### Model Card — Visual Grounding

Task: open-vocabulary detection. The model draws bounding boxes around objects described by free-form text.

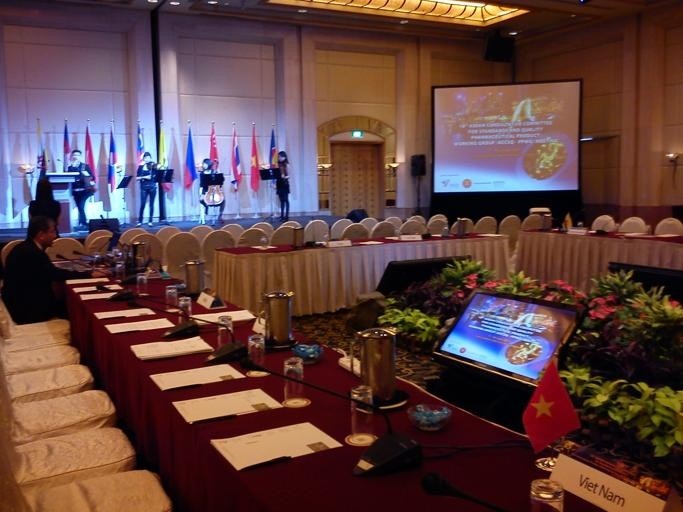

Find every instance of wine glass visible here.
[532,434,565,470]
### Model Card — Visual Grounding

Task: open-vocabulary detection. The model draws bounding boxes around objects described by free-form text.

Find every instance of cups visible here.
[282,357,306,404]
[177,297,193,322]
[135,272,148,296]
[165,283,178,315]
[113,261,124,286]
[215,314,235,349]
[349,387,375,445]
[527,477,563,511]
[244,336,264,374]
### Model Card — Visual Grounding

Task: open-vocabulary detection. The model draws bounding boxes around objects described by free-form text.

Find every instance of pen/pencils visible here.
[240,456,291,471]
[192,414,237,423]
[167,384,201,390]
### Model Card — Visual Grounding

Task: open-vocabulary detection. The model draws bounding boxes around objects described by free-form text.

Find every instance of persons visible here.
[68,149,99,230]
[196,157,227,224]
[0,217,112,324]
[275,150,292,219]
[135,150,159,228]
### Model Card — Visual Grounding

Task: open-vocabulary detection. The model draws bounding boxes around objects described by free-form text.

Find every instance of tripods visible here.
[263,179,286,226]
[120,189,136,228]
[208,181,223,228]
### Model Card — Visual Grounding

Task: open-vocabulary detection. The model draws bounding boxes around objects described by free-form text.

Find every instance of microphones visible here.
[72,249,104,266]
[55,254,92,270]
[95,284,201,339]
[239,357,421,477]
[127,301,248,364]
[56,158,74,172]
[421,470,506,510]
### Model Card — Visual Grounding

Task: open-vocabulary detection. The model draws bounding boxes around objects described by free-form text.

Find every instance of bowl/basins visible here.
[291,342,324,364]
[405,403,451,431]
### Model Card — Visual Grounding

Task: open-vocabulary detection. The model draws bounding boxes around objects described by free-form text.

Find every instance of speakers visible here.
[482,33,515,63]
[89,219,119,233]
[410,154,427,176]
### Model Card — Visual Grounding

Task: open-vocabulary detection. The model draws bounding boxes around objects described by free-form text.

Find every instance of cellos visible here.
[203,158,224,206]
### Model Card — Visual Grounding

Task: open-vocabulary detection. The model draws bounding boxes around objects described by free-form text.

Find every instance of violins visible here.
[147,161,170,170]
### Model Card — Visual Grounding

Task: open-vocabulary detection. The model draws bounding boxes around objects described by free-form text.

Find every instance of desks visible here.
[213,233,509,318]
[50,256,683,512]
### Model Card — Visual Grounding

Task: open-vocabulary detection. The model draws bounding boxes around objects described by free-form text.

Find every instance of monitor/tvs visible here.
[608,259,683,304]
[430,291,588,392]
[375,255,472,295]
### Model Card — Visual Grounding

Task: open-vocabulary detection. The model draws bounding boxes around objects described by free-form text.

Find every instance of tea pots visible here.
[347,324,399,401]
[123,237,154,270]
[178,257,206,290]
[255,289,296,341]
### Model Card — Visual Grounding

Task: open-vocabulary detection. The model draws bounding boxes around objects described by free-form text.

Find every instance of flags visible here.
[521,357,583,456]
[34,116,282,195]
